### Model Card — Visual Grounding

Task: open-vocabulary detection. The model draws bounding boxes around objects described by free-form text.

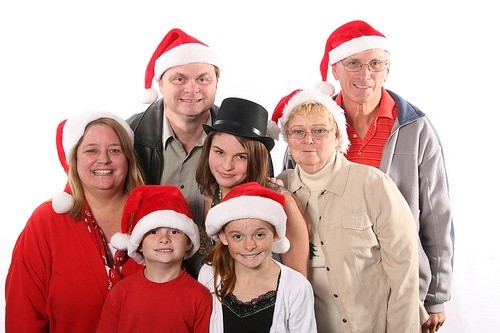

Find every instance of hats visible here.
[110,184,200,265]
[144,27,220,103]
[317,18,392,96]
[266,86,350,154]
[202,97,275,151]
[51,111,135,213]
[206,180,290,253]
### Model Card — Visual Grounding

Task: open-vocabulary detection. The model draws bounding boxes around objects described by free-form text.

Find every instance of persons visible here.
[126,36,223,221]
[4,116,146,332]
[97,185,213,333]
[192,99,312,288]
[279,22,454,333]
[195,182,318,332]
[264,87,423,333]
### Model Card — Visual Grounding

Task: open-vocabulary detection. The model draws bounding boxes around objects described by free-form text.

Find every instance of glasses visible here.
[286,127,337,139]
[341,58,389,72]
